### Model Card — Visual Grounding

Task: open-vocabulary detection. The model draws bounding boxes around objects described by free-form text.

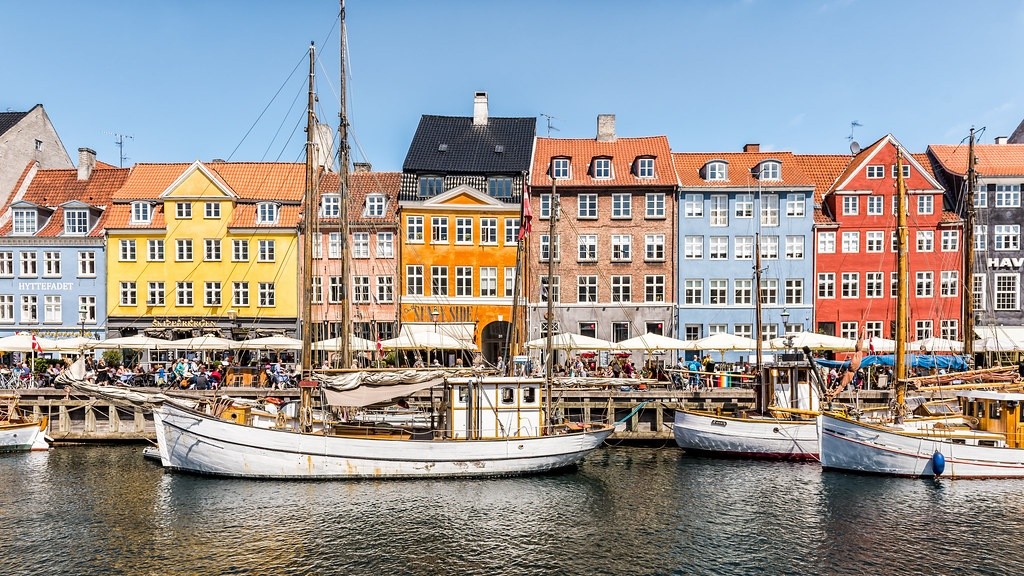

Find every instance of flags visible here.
[517,181,532,240]
[32,335,43,354]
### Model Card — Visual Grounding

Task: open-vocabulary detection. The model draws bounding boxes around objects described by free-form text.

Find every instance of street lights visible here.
[78,306,88,338]
[226,309,239,340]
[780,307,790,336]
[430,306,440,333]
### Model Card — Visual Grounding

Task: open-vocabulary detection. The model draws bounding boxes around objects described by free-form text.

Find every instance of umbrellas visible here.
[529,333,1024,363]
[0,332,480,359]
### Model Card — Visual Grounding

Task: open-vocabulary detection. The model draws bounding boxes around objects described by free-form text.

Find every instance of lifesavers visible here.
[266,396,285,404]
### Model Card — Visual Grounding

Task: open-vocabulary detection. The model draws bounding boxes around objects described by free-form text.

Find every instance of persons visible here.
[351,360,358,368]
[498,356,511,377]
[84,356,165,386]
[166,351,239,390]
[555,353,754,391]
[46,364,62,384]
[813,352,983,392]
[262,359,301,390]
[434,359,439,366]
[475,361,484,368]
[322,361,329,369]
[413,357,421,369]
[456,359,463,368]
[0,362,30,387]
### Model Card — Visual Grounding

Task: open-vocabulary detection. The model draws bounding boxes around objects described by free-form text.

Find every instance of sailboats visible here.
[139,0,628,484]
[672,122,1024,481]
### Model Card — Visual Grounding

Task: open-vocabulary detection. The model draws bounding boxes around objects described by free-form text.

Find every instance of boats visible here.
[0,393,56,453]
